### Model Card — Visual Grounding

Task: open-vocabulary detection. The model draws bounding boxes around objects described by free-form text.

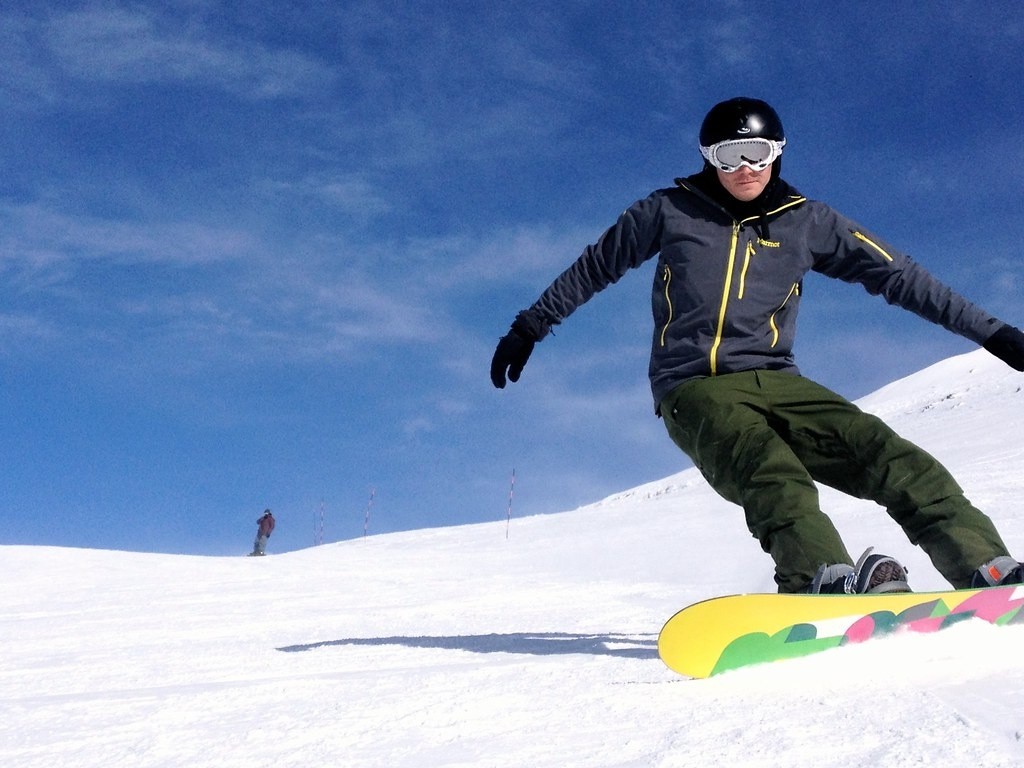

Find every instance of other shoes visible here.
[809,556,907,593]
[971,556,1024,588]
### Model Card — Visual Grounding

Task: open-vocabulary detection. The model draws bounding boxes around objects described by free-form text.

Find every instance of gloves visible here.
[983,323,1024,373]
[490,319,534,389]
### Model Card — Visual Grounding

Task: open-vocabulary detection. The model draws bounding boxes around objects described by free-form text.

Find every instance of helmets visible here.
[700,97,785,150]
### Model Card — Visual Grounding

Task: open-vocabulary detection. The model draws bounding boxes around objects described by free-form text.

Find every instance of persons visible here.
[251,509,274,555]
[488,96,1024,596]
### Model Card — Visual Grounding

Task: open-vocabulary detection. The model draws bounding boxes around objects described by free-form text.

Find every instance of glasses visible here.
[708,138,775,172]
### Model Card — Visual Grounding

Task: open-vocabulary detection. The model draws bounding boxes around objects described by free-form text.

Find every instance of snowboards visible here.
[656,582,1024,681]
